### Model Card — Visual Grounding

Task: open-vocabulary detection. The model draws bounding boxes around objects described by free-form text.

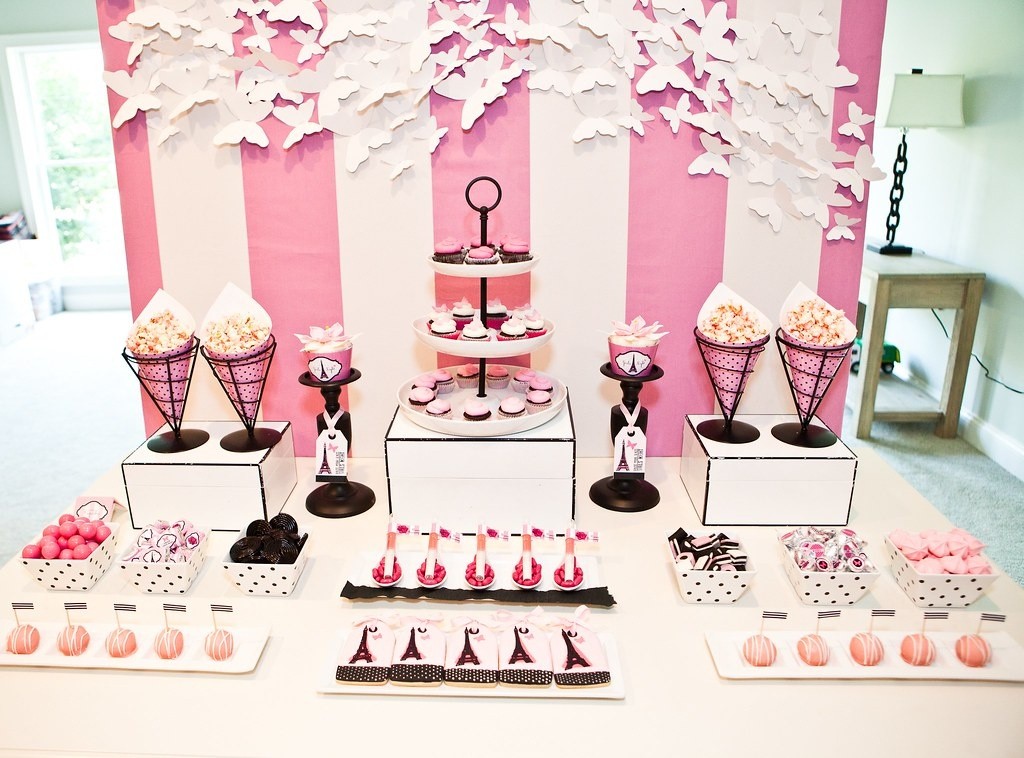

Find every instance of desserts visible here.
[608,315,669,377]
[5,625,233,661]
[22,514,309,564]
[742,633,992,668]
[667,527,992,575]
[294,323,355,382]
[409,232,556,422]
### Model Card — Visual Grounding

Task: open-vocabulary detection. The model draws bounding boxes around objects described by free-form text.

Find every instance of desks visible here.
[852,236,988,437]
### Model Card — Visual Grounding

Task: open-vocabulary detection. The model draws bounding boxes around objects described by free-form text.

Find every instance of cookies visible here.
[335,617,612,689]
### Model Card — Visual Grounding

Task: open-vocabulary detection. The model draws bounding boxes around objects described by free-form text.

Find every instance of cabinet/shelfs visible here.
[396,251,568,436]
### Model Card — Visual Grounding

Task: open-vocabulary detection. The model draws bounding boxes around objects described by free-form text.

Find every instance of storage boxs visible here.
[665,527,760,605]
[116,521,211,595]
[882,528,1002,607]
[218,525,310,597]
[778,528,879,603]
[16,522,117,590]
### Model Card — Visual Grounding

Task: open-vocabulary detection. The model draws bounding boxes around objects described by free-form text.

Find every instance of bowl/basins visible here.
[17,522,120,591]
[886,531,999,607]
[609,336,660,379]
[117,526,212,595]
[223,524,310,597]
[303,344,352,383]
[666,531,755,604]
[780,531,880,606]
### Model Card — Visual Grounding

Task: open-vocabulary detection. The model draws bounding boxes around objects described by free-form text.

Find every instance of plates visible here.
[703,630,1024,682]
[318,612,624,699]
[340,534,613,609]
[0,621,272,674]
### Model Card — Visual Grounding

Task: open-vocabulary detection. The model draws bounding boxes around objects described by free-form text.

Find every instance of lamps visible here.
[867,69,967,255]
